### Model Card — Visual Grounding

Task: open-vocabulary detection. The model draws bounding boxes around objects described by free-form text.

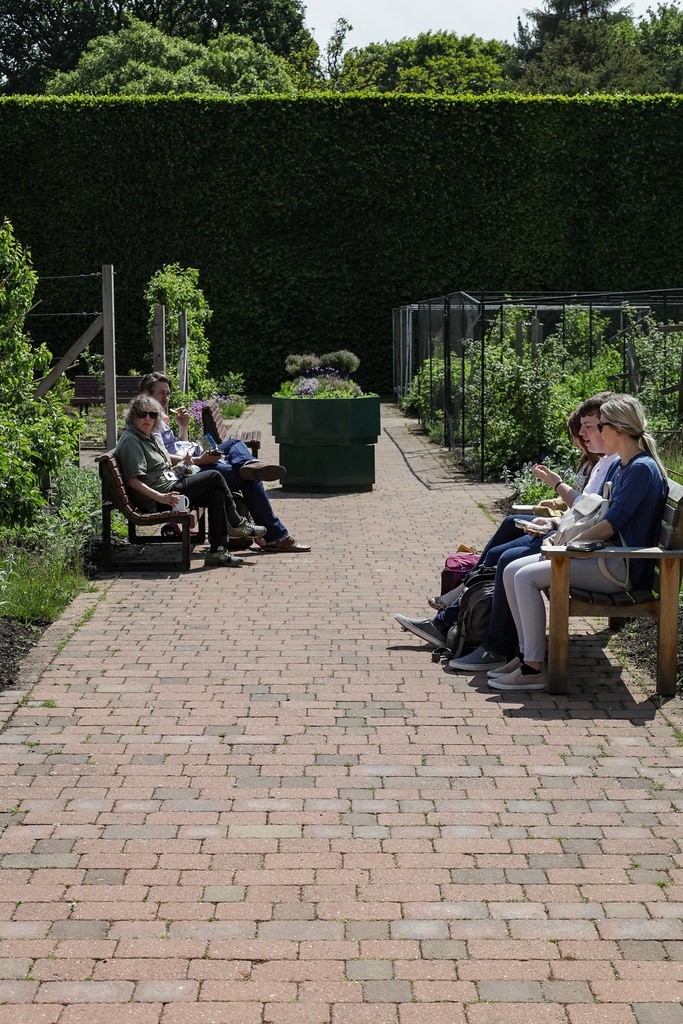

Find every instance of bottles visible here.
[447,622,458,647]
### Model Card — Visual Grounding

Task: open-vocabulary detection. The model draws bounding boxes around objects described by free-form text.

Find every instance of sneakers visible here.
[204,549,244,566]
[485,656,549,690]
[241,461,286,482]
[393,613,448,648]
[265,535,312,552]
[227,519,267,539]
[449,645,506,670]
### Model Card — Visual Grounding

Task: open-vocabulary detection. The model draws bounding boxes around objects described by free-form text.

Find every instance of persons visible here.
[116,372,312,567]
[394,391,670,690]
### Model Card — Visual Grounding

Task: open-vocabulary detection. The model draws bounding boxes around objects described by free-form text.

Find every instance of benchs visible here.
[202,400,260,459]
[94,445,204,572]
[70,376,144,418]
[510,475,683,694]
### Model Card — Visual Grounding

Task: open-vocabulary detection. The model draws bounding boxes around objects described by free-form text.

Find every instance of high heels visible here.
[428,596,453,609]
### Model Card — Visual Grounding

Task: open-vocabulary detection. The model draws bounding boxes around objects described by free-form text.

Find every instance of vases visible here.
[271,392,380,489]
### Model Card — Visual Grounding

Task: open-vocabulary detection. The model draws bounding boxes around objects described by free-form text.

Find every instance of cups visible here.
[171,495,189,511]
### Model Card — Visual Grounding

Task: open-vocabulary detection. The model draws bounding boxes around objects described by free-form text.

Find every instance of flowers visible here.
[271,349,377,398]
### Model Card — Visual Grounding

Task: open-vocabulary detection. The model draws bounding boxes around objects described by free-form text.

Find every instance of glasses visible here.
[135,408,160,419]
[597,421,615,433]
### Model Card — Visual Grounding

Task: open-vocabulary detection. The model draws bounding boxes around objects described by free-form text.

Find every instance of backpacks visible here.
[555,481,631,588]
[442,551,484,594]
[451,566,496,657]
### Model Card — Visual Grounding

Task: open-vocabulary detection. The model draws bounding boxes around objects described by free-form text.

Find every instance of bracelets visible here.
[548,536,555,545]
[555,481,565,492]
[551,518,558,531]
